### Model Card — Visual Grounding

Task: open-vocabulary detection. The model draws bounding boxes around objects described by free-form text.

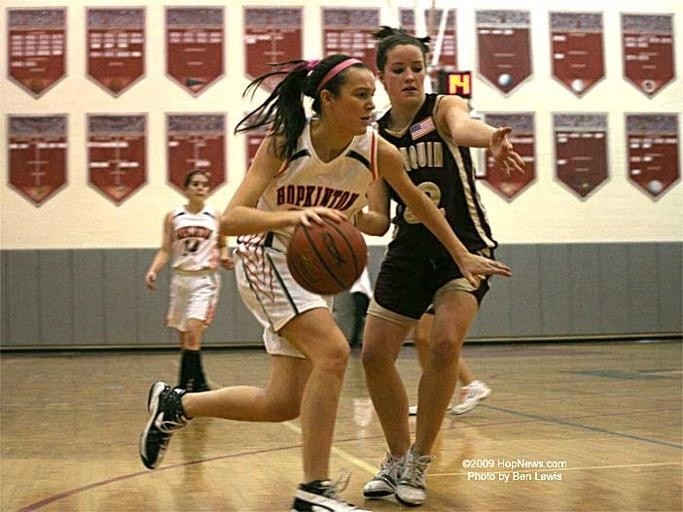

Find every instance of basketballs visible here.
[287,215,368,295]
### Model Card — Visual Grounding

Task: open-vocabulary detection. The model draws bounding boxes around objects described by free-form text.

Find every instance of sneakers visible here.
[452,380,492,415]
[292,479,362,512]
[395,443,434,506]
[140,381,192,469]
[408,402,452,416]
[363,455,404,498]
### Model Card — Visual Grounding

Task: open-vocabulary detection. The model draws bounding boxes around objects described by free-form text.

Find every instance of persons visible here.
[138,54,511,512]
[406,302,492,415]
[357,27,525,507]
[146,171,235,392]
[350,266,373,348]
